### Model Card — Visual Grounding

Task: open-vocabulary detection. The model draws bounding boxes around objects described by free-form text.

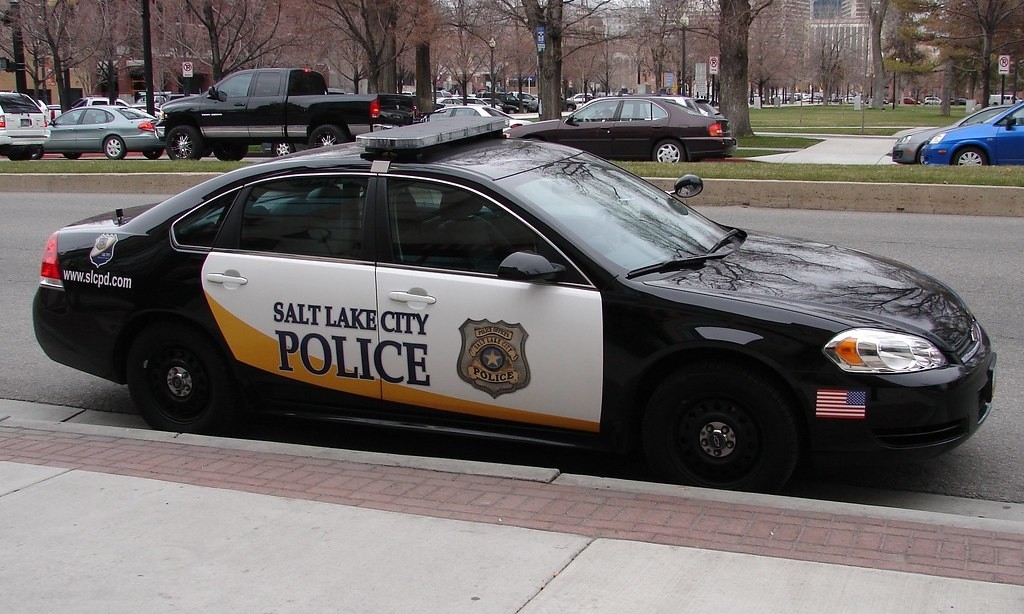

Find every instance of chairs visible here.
[439,191,514,273]
[395,192,450,267]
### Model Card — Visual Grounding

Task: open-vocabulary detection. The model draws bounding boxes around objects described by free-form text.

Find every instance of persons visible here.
[992,102,998,107]
[1004,113,1021,125]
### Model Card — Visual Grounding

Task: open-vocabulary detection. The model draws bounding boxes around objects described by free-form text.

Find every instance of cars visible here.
[42,106,166,159]
[892,105,1018,165]
[401,82,607,113]
[749,93,890,105]
[46,90,196,118]
[898,97,921,104]
[28,117,1001,501]
[921,103,1023,165]
[950,98,968,105]
[0,91,52,161]
[419,105,533,137]
[566,96,727,121]
[499,96,737,163]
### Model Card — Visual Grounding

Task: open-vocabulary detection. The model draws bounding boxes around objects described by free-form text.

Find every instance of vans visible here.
[924,97,942,105]
[984,95,1023,104]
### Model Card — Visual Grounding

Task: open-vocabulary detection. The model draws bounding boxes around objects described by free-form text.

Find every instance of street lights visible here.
[490,36,497,109]
[679,12,689,97]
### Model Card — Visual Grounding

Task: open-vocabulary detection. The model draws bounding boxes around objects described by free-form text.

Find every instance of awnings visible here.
[509,73,536,81]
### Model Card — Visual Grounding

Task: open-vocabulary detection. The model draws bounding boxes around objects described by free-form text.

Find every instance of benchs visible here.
[244,185,368,257]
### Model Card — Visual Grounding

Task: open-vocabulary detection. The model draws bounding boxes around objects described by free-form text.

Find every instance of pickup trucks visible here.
[155,68,418,161]
